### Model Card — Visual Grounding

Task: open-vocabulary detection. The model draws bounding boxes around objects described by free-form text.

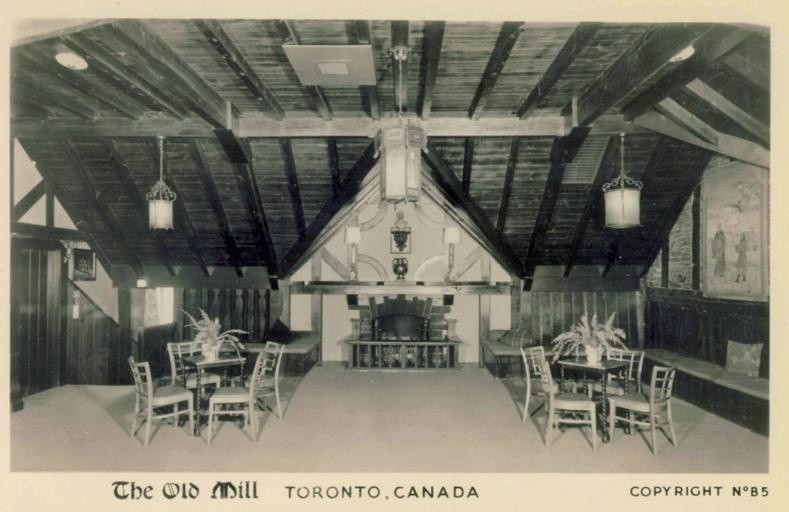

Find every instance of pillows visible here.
[500,324,535,351]
[264,319,295,345]
[722,337,765,378]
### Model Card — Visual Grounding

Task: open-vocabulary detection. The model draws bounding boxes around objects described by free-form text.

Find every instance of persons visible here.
[734,232,747,282]
[712,223,726,277]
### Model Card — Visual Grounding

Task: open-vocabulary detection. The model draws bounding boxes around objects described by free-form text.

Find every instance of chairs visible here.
[510,340,682,457]
[122,332,287,450]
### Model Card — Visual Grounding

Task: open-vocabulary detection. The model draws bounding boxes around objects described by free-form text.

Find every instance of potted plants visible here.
[551,314,630,368]
[180,307,246,362]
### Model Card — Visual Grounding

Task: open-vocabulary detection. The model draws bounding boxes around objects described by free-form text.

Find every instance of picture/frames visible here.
[696,155,770,302]
[71,246,99,283]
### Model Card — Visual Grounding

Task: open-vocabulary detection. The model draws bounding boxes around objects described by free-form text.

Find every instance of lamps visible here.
[442,227,460,282]
[372,43,429,207]
[601,130,646,231]
[344,224,362,281]
[143,130,176,234]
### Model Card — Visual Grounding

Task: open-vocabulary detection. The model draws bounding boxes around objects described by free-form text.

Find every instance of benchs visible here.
[633,292,768,439]
[479,286,641,383]
[174,324,322,377]
